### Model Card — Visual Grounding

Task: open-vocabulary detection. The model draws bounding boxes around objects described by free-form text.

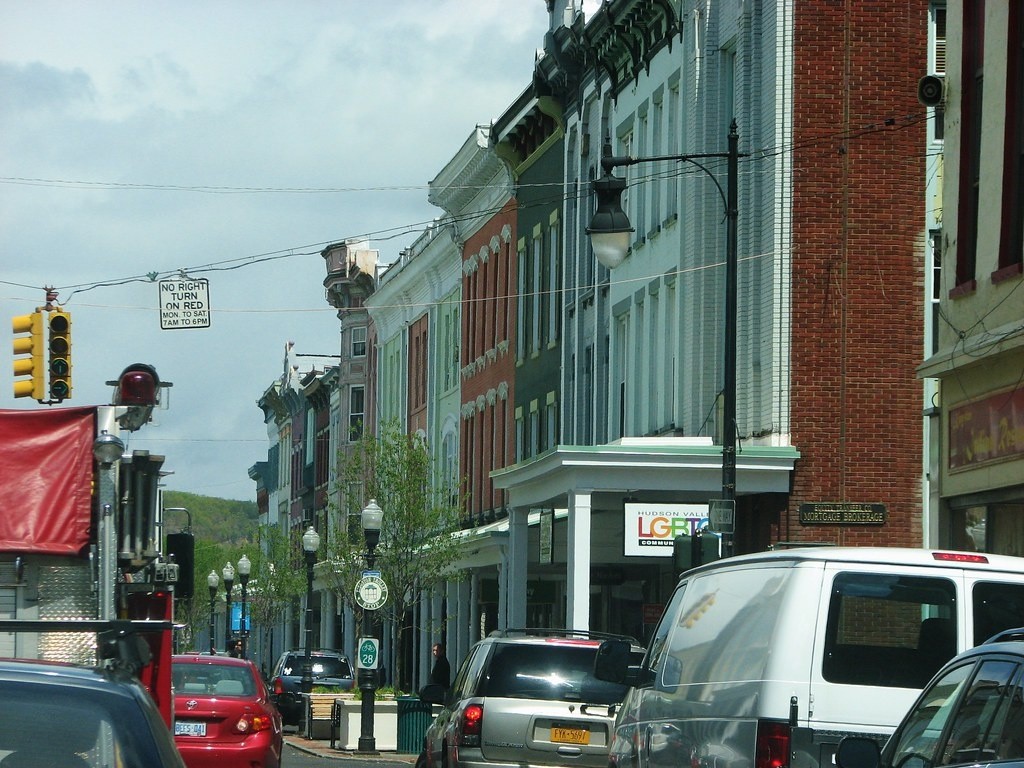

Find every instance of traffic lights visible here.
[10,311,46,401]
[45,311,76,401]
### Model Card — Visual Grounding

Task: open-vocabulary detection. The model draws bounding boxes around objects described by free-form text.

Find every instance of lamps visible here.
[92,429,126,465]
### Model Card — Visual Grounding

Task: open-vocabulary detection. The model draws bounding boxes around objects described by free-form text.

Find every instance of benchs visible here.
[299,692,411,739]
[330,699,341,749]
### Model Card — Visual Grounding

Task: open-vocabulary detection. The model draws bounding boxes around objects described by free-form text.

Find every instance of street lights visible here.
[583,115,741,563]
[205,569,219,657]
[357,499,389,757]
[221,561,235,657]
[299,525,320,737]
[235,554,252,658]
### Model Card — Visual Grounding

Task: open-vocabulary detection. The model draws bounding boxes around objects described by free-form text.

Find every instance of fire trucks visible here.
[0,363,197,727]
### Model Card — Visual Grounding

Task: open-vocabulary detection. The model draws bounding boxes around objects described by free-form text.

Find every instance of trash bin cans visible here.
[393,696,433,754]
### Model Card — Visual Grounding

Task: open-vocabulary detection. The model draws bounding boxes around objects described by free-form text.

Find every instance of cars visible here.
[146,654,285,768]
[0,656,190,768]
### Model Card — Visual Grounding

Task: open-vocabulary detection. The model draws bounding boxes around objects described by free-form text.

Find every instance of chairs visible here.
[214,679,245,696]
[918,617,953,656]
[0,749,91,768]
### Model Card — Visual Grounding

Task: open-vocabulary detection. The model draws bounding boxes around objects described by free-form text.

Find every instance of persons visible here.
[432,643,450,686]
[379,664,386,688]
[222,637,241,658]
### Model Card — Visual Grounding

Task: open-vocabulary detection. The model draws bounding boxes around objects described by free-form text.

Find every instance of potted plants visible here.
[298,686,410,738]
[339,691,420,751]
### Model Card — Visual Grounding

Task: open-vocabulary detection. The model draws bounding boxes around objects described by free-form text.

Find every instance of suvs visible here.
[413,626,650,767]
[594,544,1024,768]
[836,627,1024,764]
[269,650,354,725]
